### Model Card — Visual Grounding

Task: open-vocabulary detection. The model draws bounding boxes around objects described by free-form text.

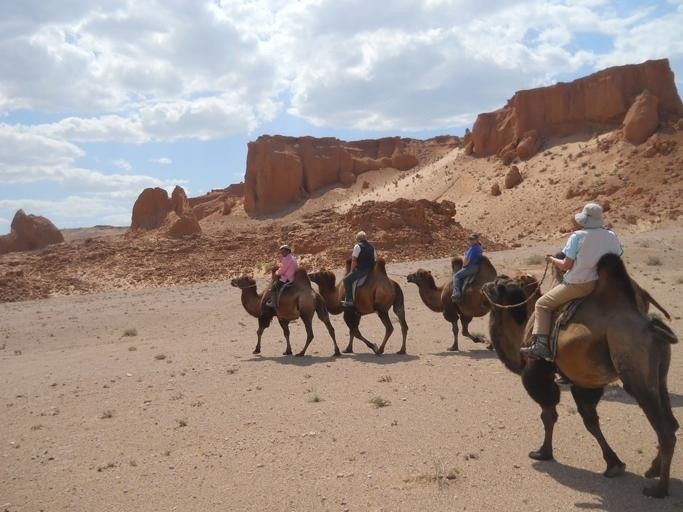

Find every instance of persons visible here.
[337,230,377,308]
[448,233,482,300]
[263,244,298,308]
[517,202,624,363]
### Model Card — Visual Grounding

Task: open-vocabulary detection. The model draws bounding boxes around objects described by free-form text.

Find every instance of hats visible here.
[575,204,603,228]
[469,234,480,239]
[280,244,291,250]
[355,231,368,242]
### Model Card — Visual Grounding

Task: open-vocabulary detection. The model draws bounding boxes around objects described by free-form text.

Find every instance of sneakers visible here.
[452,293,460,298]
[520,343,551,357]
[339,298,351,306]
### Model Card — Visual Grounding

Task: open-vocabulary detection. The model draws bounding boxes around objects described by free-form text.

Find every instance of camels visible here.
[479,251,680,499]
[230,265,343,357]
[406,255,497,351]
[306,256,409,355]
